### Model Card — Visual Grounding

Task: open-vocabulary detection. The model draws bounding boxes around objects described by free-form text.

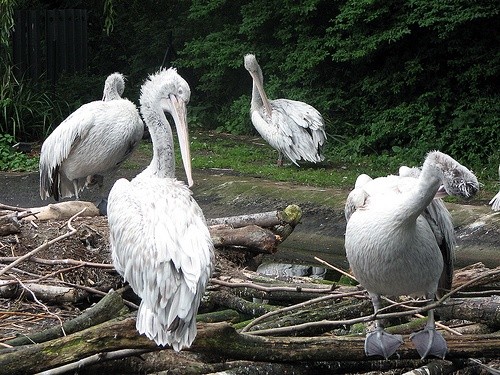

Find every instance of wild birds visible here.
[243,53,327,169]
[344,149,499,361]
[106,66,215,352]
[39,72,144,201]
